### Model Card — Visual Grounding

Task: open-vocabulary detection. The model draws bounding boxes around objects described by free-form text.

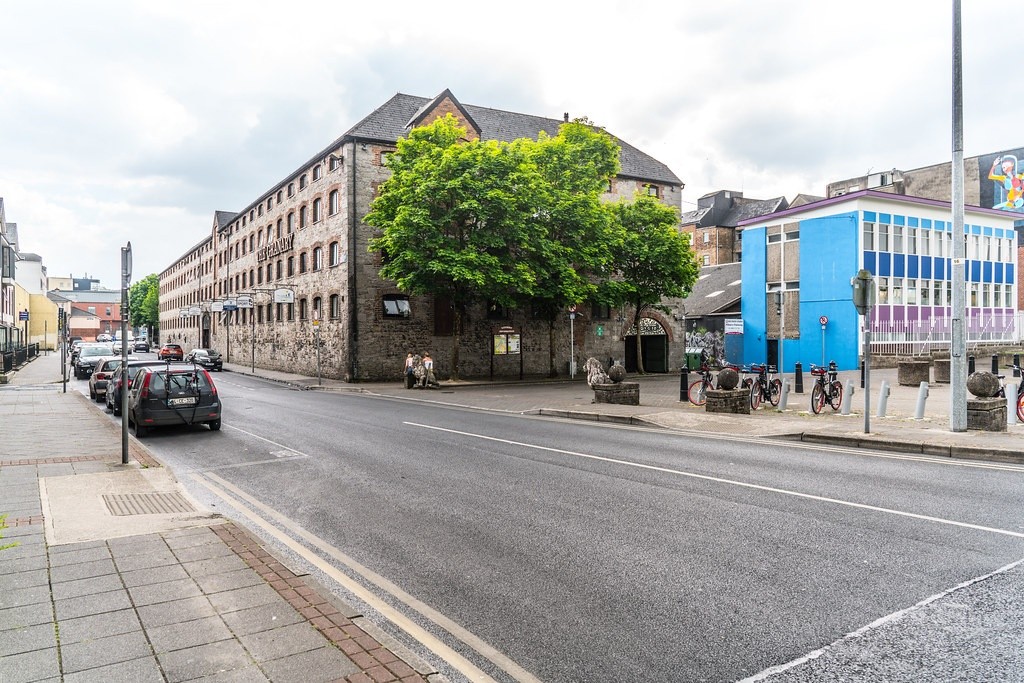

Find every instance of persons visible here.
[423,352,433,371]
[697,349,711,404]
[405,353,416,389]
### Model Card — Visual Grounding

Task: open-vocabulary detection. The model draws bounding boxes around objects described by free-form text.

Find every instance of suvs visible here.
[68,336,82,348]
[158,343,183,360]
[128,365,222,437]
[87,356,138,403]
[105,361,168,413]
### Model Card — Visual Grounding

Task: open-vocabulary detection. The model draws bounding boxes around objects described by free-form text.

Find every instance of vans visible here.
[115,330,134,341]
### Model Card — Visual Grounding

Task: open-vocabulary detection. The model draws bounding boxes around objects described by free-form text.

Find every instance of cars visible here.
[97,334,114,342]
[135,340,149,353]
[69,340,114,380]
[114,342,132,354]
[186,349,224,370]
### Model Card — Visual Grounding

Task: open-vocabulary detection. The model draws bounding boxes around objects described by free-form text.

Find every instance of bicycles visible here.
[1005,363,1024,422]
[810,362,843,414]
[733,363,782,411]
[689,364,716,407]
